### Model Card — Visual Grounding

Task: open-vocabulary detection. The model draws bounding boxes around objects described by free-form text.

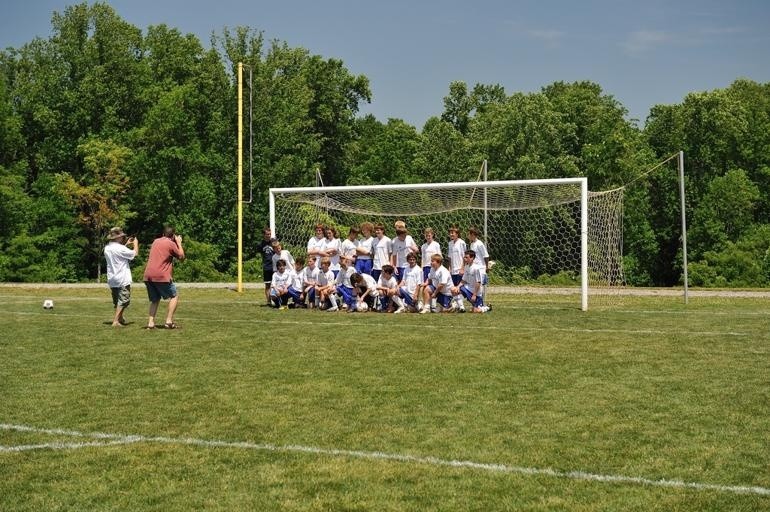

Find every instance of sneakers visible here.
[459,304,466,313]
[278,304,288,310]
[420,308,430,314]
[486,303,492,311]
[328,305,339,312]
[394,305,405,313]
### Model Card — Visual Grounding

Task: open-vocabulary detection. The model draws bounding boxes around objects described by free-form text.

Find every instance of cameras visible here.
[174,234,183,242]
[130,238,135,242]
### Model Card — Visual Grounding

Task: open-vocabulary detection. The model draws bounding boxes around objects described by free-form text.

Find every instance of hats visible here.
[107,227,127,240]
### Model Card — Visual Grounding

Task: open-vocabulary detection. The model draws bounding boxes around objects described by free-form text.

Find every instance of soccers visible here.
[357,301,368,312]
[43,299,53,310]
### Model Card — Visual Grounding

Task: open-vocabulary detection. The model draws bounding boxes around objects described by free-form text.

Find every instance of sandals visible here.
[164,322,178,329]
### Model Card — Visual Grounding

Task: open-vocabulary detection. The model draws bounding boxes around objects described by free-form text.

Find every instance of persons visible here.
[102,227,139,329]
[258,220,493,315]
[141,226,186,330]
